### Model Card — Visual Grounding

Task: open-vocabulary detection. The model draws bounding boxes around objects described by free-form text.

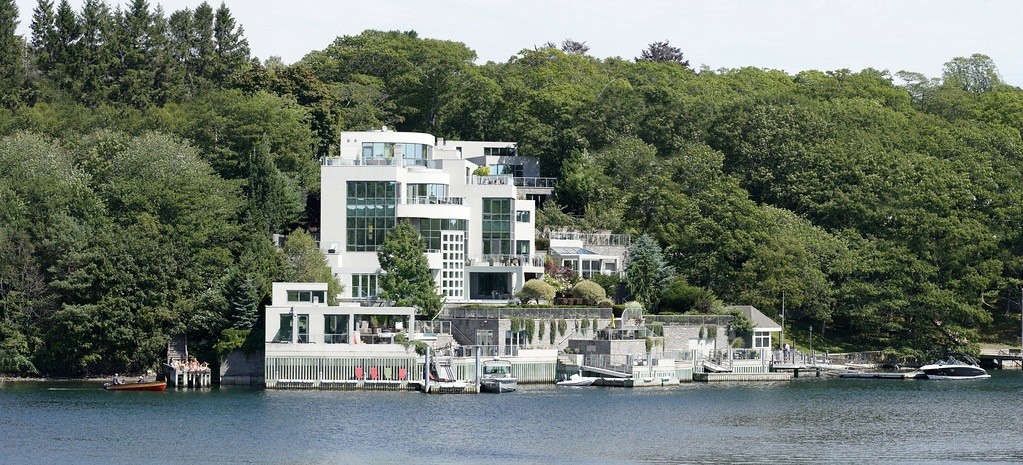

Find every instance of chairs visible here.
[355,368,363,380]
[384,368,392,380]
[399,369,407,380]
[483,179,491,184]
[370,368,379,381]
[499,256,517,267]
[494,179,502,185]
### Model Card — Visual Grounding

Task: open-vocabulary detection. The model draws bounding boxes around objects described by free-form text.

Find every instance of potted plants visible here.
[466,258,478,266]
[532,257,543,267]
[487,257,496,266]
[518,255,525,266]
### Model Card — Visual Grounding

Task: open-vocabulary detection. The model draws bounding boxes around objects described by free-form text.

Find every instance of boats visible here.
[895,364,928,379]
[919,352,992,380]
[103,381,168,392]
[480,345,518,393]
[555,373,597,386]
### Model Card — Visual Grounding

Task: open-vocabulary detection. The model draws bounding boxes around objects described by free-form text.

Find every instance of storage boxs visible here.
[374,326,381,334]
[360,328,373,333]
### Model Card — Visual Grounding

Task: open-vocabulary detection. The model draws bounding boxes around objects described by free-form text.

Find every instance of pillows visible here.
[495,180,500,184]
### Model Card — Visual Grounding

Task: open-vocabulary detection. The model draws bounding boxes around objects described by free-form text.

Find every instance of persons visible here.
[113,373,125,385]
[138,376,145,384]
